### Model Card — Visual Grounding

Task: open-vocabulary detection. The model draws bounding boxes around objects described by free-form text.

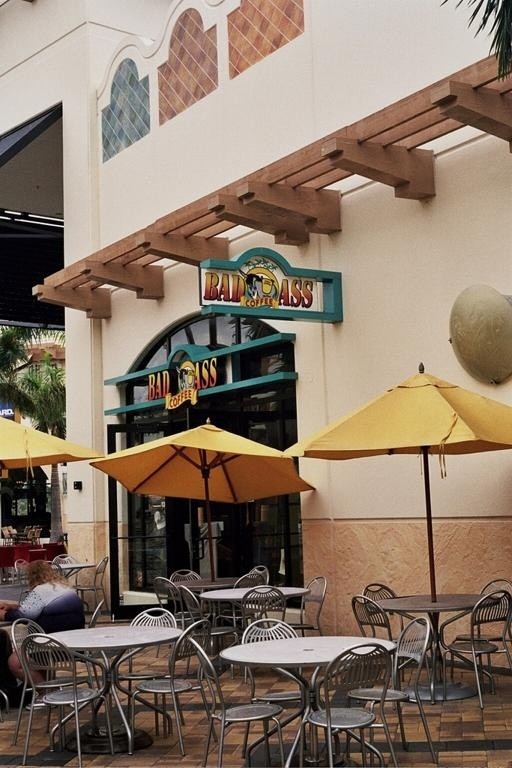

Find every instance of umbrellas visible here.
[283,364,512,684]
[89,418,315,654]
[0,416,104,469]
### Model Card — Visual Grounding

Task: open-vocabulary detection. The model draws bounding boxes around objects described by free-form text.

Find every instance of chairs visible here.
[10,618,97,745]
[351,594,434,706]
[347,616,438,765]
[186,635,288,766]
[362,583,440,677]
[300,642,397,766]
[20,631,115,765]
[1,523,328,636]
[451,578,510,696]
[241,618,315,759]
[441,591,510,711]
[115,608,185,734]
[128,619,219,755]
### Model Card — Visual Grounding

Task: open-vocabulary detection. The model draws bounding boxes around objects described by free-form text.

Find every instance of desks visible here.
[218,634,396,765]
[34,625,186,742]
[366,592,504,703]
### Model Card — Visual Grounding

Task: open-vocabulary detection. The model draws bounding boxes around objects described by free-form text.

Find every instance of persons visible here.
[0,559,85,710]
